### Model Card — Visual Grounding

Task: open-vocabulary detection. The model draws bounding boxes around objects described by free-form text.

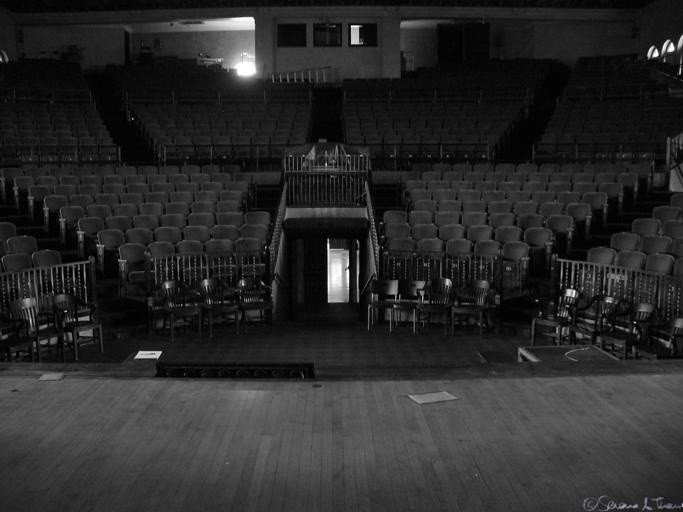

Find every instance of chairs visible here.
[4,99,661,365]
